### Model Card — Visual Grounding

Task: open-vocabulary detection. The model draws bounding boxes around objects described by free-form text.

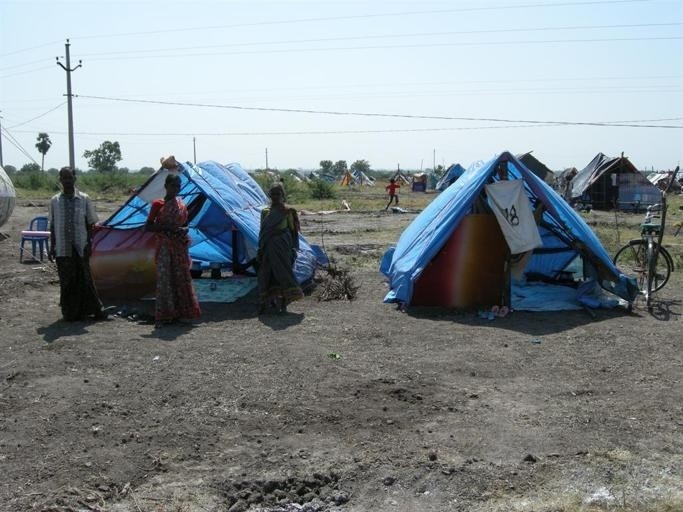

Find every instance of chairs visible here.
[20,216,55,264]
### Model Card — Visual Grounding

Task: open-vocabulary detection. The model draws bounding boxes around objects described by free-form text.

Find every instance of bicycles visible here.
[612,165,681,313]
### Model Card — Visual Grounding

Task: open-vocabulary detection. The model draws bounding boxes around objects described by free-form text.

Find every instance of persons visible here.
[47,166,116,323]
[385,179,400,209]
[256,182,304,314]
[144,174,201,328]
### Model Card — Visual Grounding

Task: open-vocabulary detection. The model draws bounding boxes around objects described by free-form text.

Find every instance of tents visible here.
[100,155,329,286]
[434,153,683,212]
[340,169,376,187]
[389,170,410,185]
[379,151,639,311]
